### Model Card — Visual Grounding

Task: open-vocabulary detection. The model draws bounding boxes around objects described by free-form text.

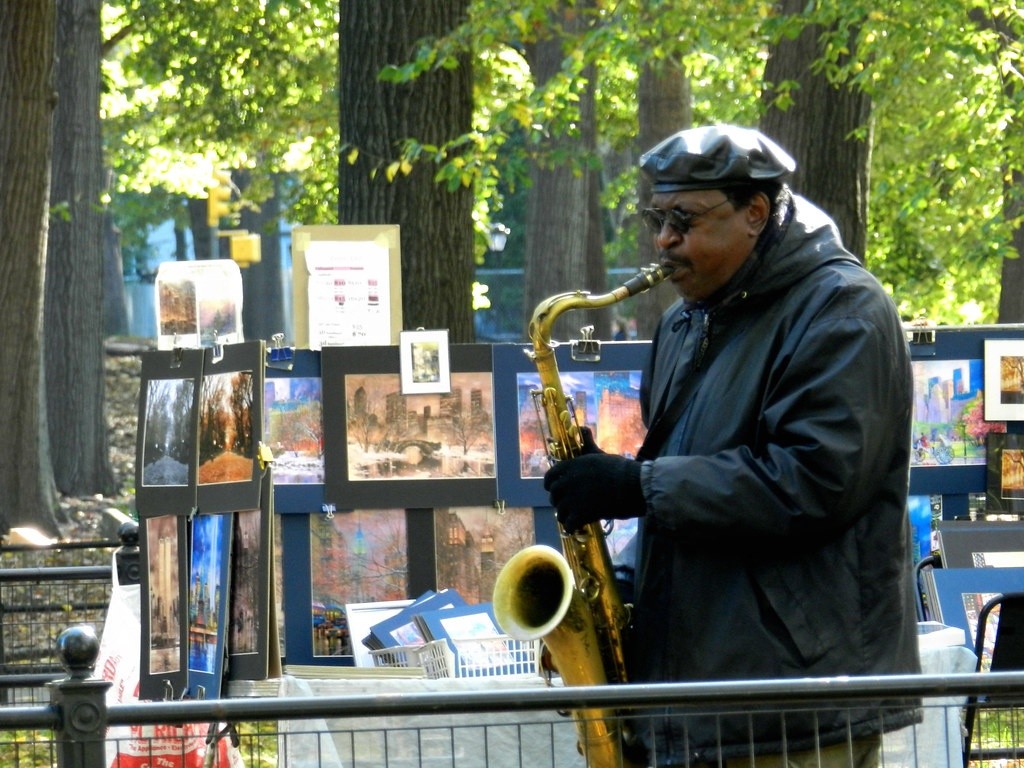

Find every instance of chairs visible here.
[962,592,1024,768]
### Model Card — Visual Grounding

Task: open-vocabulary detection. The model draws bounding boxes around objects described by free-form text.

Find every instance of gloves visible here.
[544,424,646,533]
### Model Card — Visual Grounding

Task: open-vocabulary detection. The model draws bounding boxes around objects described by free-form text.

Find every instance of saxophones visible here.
[491,257,682,768]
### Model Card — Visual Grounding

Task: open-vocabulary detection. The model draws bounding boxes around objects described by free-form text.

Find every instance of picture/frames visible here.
[984,337,1024,421]
[987,432,1024,515]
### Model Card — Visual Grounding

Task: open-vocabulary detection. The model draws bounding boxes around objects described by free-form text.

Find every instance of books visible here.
[923,518,1024,704]
[360,585,527,679]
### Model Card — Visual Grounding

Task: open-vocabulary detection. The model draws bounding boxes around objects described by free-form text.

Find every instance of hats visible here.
[638,125,797,191]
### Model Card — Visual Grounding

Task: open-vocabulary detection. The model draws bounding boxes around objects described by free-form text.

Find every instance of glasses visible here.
[641,191,746,235]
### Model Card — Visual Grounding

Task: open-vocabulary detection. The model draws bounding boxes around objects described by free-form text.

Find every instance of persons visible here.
[542,125,924,768]
